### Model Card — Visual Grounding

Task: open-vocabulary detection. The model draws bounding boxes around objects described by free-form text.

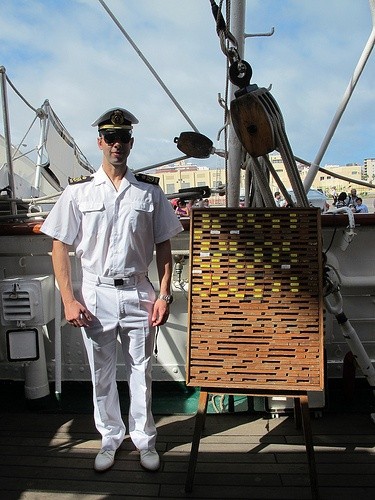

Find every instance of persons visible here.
[239,196,252,208]
[274,192,291,207]
[332,187,369,214]
[171,197,209,217]
[39,107,184,474]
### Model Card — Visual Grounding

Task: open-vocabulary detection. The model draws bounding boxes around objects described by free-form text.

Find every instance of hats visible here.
[90,107,139,134]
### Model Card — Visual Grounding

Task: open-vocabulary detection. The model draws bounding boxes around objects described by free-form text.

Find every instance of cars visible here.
[279,188,330,212]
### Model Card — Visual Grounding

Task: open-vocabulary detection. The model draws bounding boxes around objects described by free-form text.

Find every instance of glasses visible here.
[97,131,134,145]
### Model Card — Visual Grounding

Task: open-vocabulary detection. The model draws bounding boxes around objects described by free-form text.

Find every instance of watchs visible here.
[157,294,173,304]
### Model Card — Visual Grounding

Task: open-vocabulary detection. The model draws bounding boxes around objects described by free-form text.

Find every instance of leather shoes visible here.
[140,448,160,470]
[94,448,115,471]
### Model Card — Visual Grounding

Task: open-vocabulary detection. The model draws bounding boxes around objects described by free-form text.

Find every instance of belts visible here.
[83,270,148,287]
